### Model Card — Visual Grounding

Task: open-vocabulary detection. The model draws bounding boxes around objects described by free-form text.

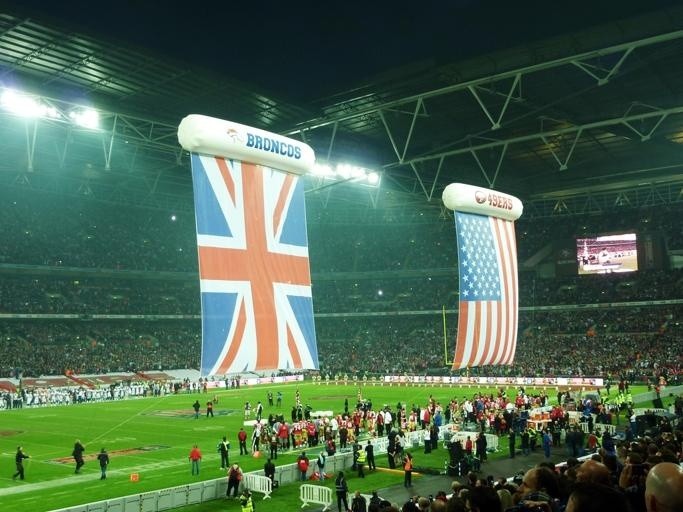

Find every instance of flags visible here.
[190,153,321,378]
